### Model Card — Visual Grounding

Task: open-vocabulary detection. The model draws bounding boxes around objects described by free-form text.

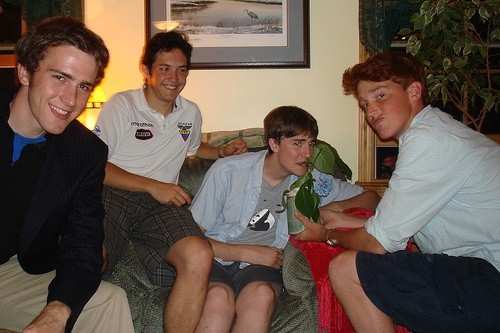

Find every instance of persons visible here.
[92,28,247,333]
[294,52,500,333]
[188,106,382,333]
[0,16,136,333]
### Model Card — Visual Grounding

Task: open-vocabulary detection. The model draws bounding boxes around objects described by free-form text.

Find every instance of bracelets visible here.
[218,147,224,158]
[324,228,337,246]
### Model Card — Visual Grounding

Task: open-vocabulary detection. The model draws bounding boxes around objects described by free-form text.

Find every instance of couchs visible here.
[98,128,349,333]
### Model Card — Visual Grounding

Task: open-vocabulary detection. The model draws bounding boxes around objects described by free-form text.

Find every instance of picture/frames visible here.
[375,146,399,179]
[0,0,85,70]
[145,0,311,70]
